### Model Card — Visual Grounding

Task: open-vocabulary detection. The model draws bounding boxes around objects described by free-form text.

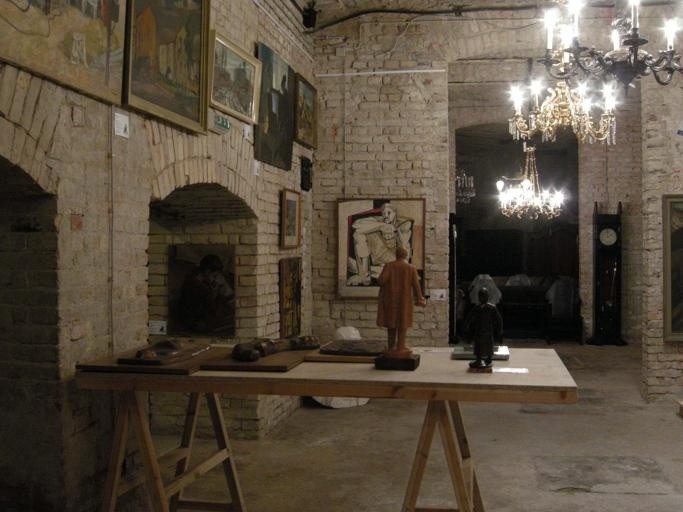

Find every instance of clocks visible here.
[587,201,626,344]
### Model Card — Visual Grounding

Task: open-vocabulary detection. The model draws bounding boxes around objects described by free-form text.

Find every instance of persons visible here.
[377,246,424,353]
[465,287,501,365]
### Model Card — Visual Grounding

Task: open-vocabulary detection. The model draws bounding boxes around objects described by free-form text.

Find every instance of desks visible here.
[72,342,578,512]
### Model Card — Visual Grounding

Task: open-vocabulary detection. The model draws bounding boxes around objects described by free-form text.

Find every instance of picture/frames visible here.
[124,1,209,136]
[294,72,319,152]
[279,189,302,251]
[333,197,425,304]
[662,192,682,344]
[209,28,264,125]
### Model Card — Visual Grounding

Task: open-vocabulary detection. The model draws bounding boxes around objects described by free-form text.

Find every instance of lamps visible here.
[492,139,563,222]
[502,58,621,146]
[454,168,476,205]
[533,0,683,87]
